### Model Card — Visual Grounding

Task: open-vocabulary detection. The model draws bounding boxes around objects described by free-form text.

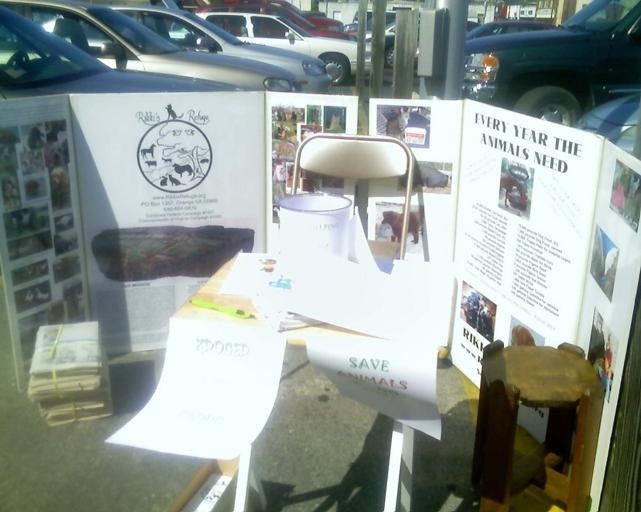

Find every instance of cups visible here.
[276,194,352,263]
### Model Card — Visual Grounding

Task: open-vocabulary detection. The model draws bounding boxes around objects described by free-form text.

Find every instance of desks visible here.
[159,248,458,510]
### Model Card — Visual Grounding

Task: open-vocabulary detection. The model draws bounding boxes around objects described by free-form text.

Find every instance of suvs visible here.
[463,1,641,127]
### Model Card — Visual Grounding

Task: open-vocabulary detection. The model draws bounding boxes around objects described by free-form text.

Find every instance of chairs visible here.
[286,134,419,268]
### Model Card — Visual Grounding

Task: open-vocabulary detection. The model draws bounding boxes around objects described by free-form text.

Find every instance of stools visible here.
[469,336,606,510]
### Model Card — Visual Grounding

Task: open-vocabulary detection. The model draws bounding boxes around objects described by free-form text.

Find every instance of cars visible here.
[501,163,530,211]
[466,15,556,38]
[576,89,640,159]
